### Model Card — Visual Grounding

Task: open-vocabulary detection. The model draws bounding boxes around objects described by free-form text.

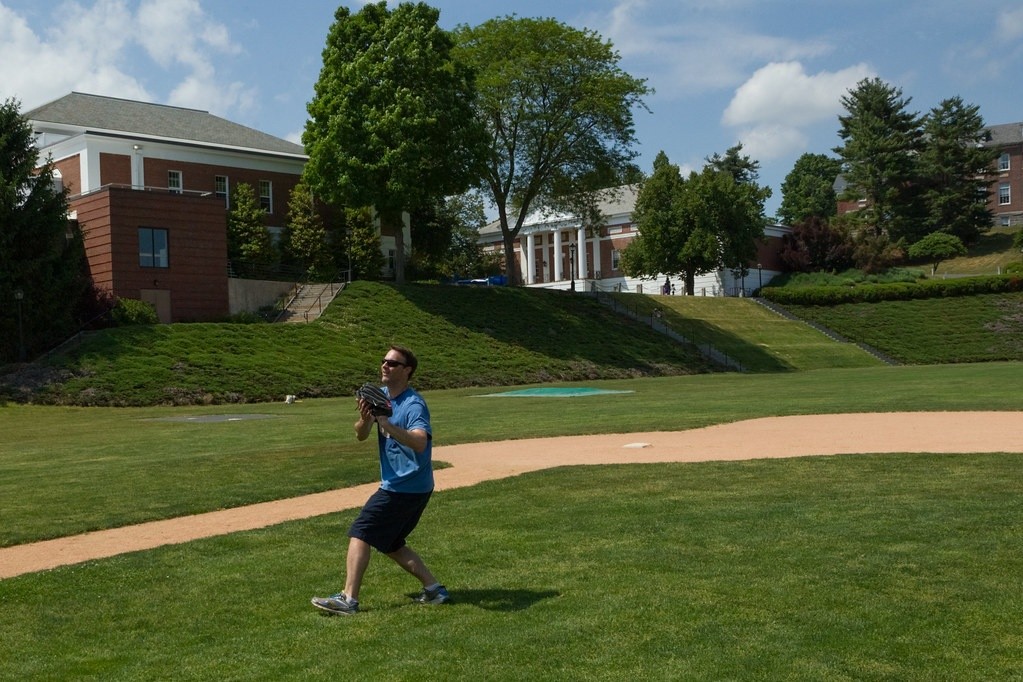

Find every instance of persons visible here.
[310,346,450,615]
[671,284,675,295]
[665,278,671,295]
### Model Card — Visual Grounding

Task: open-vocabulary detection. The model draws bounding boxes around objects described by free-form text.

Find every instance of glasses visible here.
[382,359,406,369]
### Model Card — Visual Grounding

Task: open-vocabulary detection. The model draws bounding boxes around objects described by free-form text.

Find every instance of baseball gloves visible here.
[356,381,393,417]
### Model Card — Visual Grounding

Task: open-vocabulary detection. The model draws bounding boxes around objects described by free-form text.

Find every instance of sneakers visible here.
[416,585,449,604]
[311,592,359,616]
